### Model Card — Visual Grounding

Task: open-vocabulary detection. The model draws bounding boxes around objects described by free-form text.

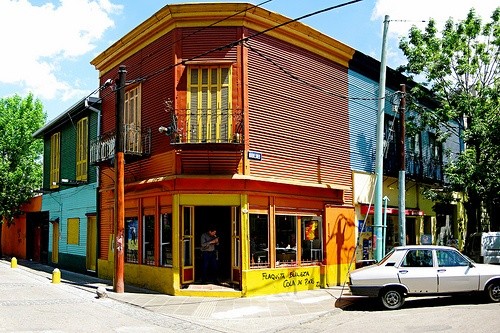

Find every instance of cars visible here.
[345,245,500,310]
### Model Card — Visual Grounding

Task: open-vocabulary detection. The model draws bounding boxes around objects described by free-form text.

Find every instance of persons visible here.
[199,227,225,286]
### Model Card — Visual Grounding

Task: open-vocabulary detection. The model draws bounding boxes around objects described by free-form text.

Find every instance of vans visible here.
[465,231,500,265]
